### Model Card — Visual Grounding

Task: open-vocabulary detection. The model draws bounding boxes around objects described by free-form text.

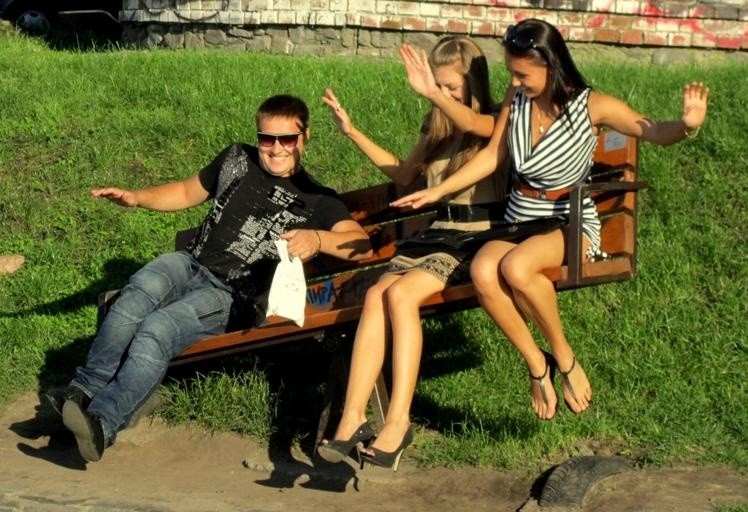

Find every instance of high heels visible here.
[317,423,374,470]
[359,426,414,472]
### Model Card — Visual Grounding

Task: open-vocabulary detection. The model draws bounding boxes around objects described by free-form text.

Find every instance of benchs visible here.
[97,128,638,477]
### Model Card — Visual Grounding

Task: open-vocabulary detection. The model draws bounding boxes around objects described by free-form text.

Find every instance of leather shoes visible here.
[63,399,105,463]
[44,385,87,416]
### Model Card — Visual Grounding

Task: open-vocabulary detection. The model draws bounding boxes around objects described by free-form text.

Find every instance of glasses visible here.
[257,131,304,147]
[503,24,538,50]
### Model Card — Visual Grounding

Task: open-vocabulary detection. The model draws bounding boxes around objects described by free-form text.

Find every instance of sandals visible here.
[556,355,592,414]
[526,348,560,420]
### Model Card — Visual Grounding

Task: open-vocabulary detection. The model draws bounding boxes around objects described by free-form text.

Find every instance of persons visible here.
[42,92,376,464]
[315,32,513,473]
[387,17,712,424]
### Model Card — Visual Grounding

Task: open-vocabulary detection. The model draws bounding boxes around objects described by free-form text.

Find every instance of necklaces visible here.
[530,100,558,136]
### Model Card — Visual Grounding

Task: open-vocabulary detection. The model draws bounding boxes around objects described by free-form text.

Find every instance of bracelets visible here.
[312,228,322,257]
[679,117,701,140]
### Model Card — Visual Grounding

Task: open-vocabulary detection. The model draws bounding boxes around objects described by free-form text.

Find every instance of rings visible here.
[337,104,340,107]
